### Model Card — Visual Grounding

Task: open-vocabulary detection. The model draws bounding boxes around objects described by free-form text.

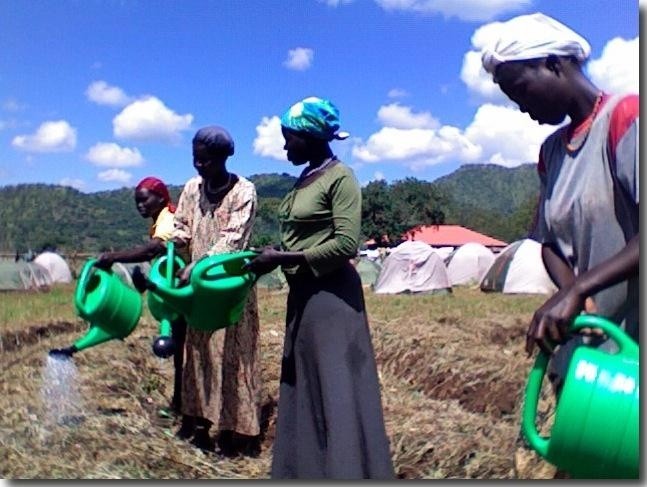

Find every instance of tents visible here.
[344,246,391,285]
[14,248,71,288]
[481,237,560,294]
[366,223,509,248]
[433,244,455,263]
[448,243,495,286]
[254,266,287,288]
[374,241,452,295]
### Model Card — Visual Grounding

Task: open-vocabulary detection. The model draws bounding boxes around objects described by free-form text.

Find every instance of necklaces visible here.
[300,155,335,179]
[205,172,231,194]
[558,90,601,150]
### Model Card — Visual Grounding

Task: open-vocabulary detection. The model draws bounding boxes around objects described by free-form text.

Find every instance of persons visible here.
[472,12,639,478]
[160,124,260,445]
[241,95,396,482]
[97,176,191,418]
